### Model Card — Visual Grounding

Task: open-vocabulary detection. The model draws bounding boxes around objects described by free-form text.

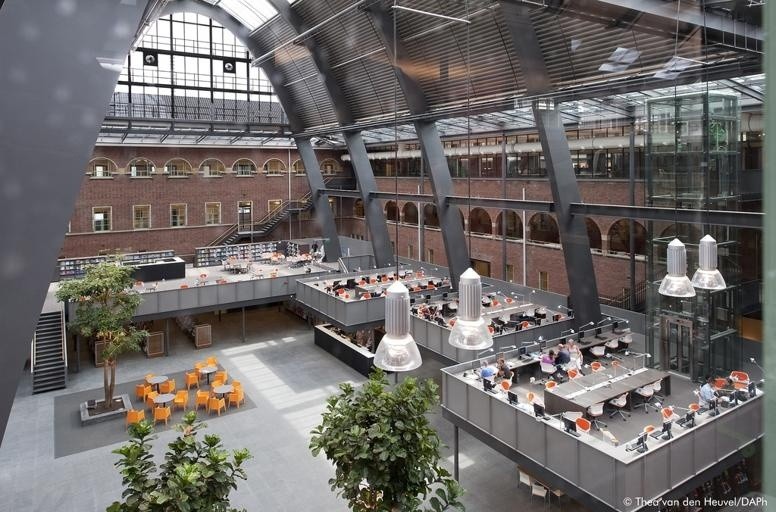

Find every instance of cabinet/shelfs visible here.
[60,240,276,281]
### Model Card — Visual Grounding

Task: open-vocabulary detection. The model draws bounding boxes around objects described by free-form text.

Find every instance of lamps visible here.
[373,0,421,372]
[658,0,695,296]
[447,0,494,351]
[691,0,727,293]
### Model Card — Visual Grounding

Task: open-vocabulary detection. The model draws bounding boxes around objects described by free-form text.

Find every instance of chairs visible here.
[127,253,758,510]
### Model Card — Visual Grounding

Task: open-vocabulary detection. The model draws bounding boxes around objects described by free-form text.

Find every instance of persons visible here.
[724,377,734,390]
[540,349,555,366]
[550,344,570,383]
[697,379,716,407]
[480,359,495,393]
[496,359,511,380]
[567,339,586,376]
[701,374,712,390]
